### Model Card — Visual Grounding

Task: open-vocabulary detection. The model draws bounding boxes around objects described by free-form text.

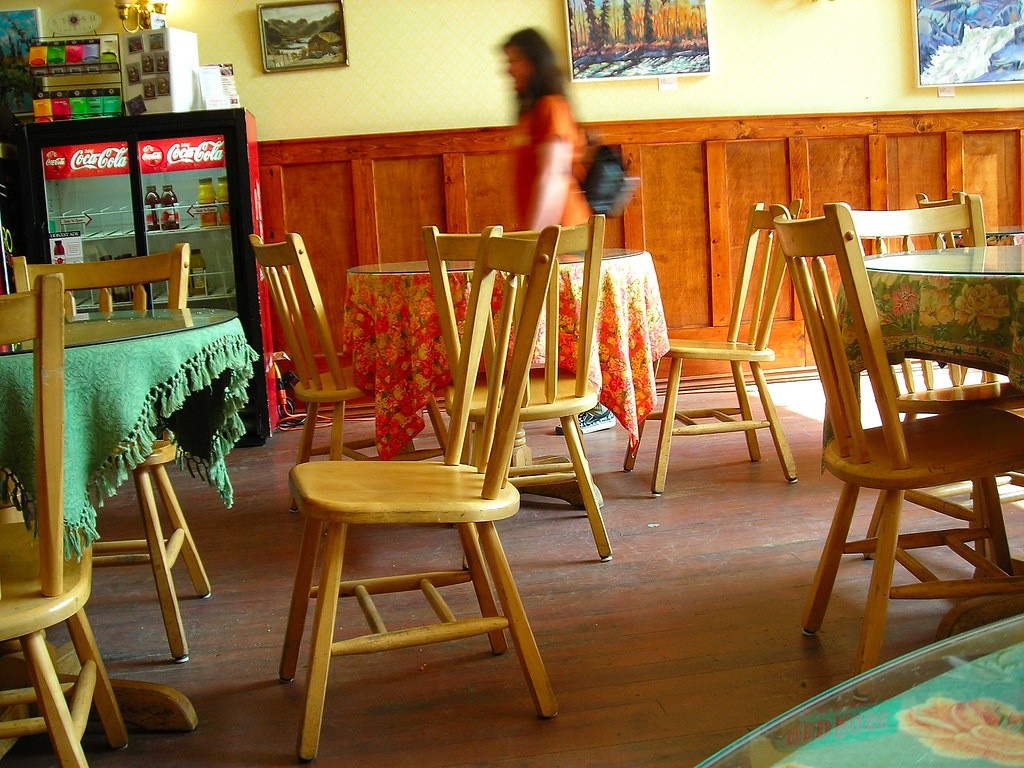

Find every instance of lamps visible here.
[114,0,168,33]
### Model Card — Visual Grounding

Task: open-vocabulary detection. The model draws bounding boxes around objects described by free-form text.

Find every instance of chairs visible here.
[0,191,1024,768]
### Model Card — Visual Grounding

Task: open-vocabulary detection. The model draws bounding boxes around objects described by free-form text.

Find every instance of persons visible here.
[503,29,617,436]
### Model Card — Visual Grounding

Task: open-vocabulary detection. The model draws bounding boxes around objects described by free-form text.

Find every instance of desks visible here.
[942,225,1024,250]
[820,245,1024,474]
[0,309,261,760]
[343,249,671,508]
[696,612,1024,767]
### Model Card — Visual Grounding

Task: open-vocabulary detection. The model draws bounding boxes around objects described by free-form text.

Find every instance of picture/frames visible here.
[911,0,1024,88]
[256,0,350,73]
[562,0,712,82]
[0,7,49,117]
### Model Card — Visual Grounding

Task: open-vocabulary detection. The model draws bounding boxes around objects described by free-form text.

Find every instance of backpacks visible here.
[535,94,635,219]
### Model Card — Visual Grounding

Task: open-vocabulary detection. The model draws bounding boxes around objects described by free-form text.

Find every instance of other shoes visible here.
[555,401,616,436]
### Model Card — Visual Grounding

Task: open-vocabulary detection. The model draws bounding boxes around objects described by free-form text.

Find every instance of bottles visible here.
[54,240,65,264]
[197,177,218,228]
[144,184,161,231]
[216,175,230,225]
[190,249,208,295]
[160,185,179,230]
